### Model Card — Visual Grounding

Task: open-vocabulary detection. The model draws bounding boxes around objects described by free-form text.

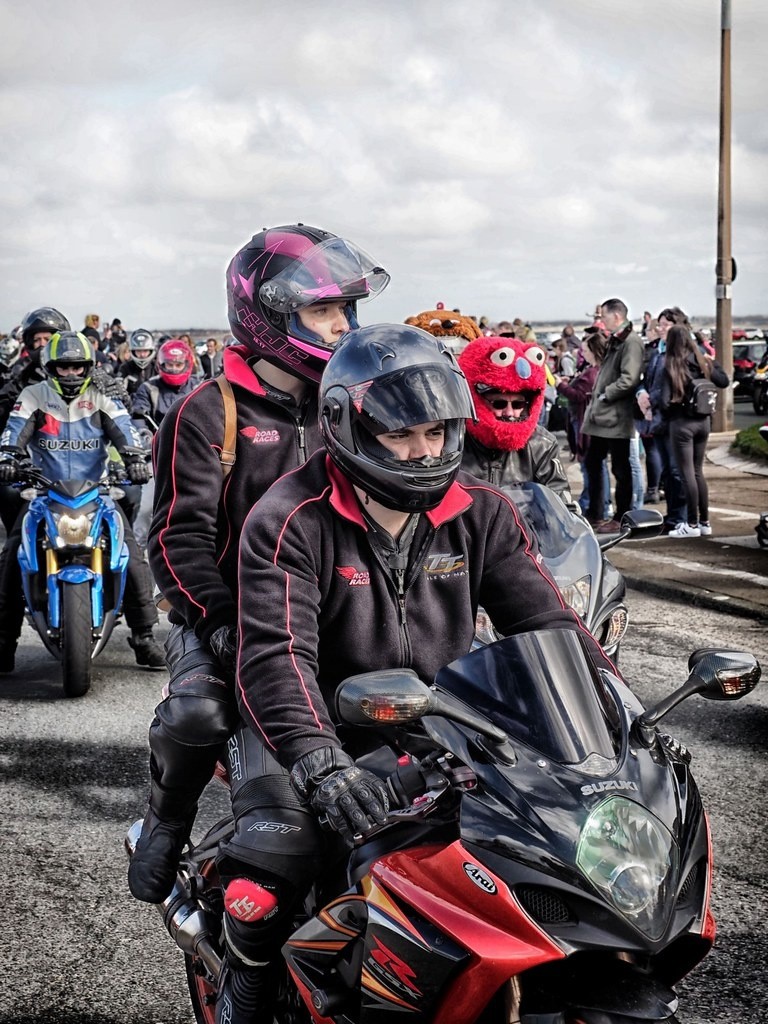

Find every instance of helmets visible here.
[318,322,479,514]
[12,326,25,349]
[227,222,371,386]
[0,338,21,368]
[157,340,192,385]
[458,336,546,449]
[129,328,156,369]
[40,330,95,398]
[22,307,71,364]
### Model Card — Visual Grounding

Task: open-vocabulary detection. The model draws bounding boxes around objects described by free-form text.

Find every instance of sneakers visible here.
[669,522,700,538]
[0,642,17,672]
[127,636,166,666]
[698,523,711,534]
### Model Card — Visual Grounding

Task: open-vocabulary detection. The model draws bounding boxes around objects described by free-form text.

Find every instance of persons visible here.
[0,307,243,681]
[405,298,730,557]
[129,224,390,904]
[213,324,631,1024]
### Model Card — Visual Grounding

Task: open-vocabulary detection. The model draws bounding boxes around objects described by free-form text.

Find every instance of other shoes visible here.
[598,520,620,532]
[644,490,665,504]
[215,921,280,1024]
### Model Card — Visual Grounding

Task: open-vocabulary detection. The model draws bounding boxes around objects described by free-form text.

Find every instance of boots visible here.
[128,753,216,903]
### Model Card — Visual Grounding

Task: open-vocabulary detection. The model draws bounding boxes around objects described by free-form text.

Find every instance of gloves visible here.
[125,456,150,485]
[288,745,388,838]
[0,451,19,486]
[656,733,691,762]
[209,625,236,674]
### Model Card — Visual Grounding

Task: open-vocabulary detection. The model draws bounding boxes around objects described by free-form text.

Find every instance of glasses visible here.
[485,397,529,409]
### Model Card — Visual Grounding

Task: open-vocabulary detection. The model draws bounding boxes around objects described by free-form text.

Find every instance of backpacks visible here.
[684,372,718,414]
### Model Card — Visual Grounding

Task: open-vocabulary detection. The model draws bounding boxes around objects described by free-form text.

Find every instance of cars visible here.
[733,344,767,401]
[734,329,765,341]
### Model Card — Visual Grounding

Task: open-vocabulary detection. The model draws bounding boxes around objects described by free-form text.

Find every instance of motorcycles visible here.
[752,358,768,414]
[124,479,762,1024]
[1,447,152,697]
